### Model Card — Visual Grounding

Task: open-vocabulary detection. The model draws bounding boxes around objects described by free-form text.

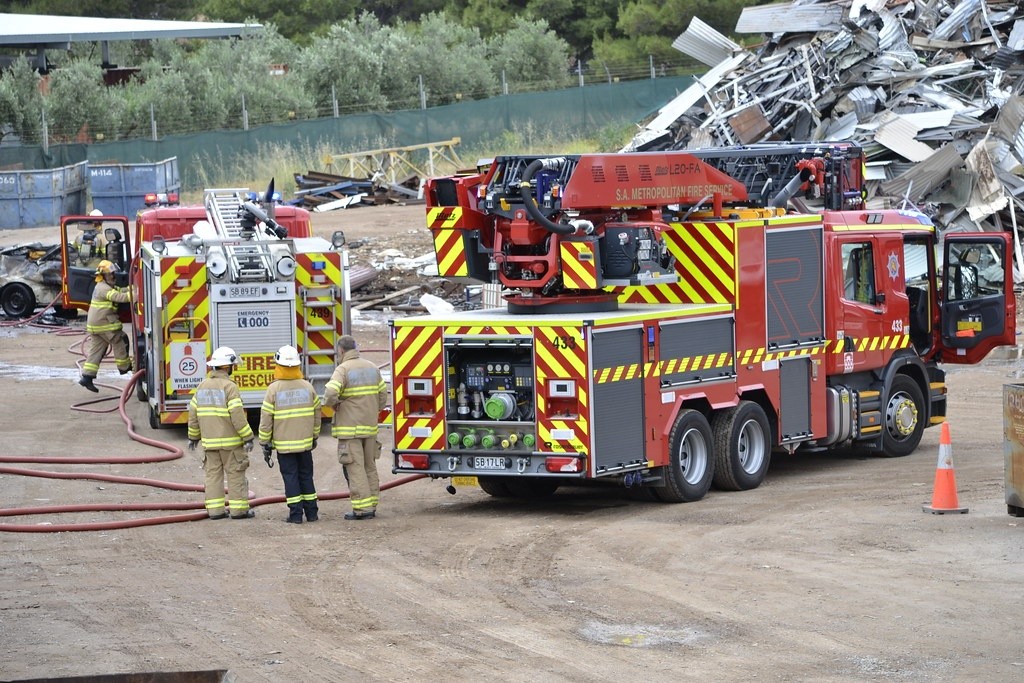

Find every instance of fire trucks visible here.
[389,136,1015,506]
[66,189,354,431]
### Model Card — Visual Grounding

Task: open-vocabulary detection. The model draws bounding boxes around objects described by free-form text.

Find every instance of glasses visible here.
[93,220,103,225]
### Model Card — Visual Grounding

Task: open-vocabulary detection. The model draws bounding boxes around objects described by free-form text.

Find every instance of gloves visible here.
[188,440,198,450]
[312,440,317,450]
[244,441,255,453]
[262,446,269,456]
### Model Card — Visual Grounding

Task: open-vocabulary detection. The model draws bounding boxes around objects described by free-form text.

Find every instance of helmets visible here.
[274,345,301,367]
[88,209,103,216]
[206,347,238,366]
[95,260,116,274]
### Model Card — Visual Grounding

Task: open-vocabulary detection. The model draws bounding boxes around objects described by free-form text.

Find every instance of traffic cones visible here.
[923,421,969,515]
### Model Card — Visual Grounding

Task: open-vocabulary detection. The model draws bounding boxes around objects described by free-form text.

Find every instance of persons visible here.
[78,260,134,393]
[259,345,321,524]
[188,347,256,520]
[323,335,387,520]
[74,208,106,258]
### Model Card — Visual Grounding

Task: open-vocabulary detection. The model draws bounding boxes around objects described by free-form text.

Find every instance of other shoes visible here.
[79,378,98,393]
[287,517,302,524]
[119,364,132,374]
[210,511,229,519]
[307,515,318,521]
[344,509,375,519]
[232,509,255,519]
